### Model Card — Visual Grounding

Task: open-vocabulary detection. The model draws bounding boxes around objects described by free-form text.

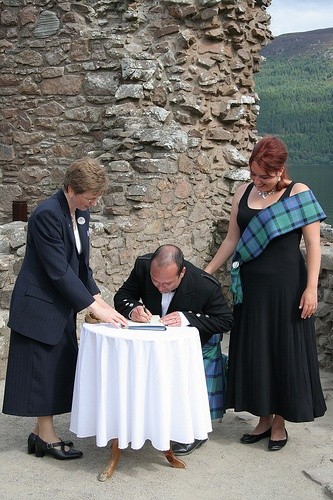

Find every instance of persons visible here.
[113,245,235,456]
[203,136,327,450]
[2,157,127,460]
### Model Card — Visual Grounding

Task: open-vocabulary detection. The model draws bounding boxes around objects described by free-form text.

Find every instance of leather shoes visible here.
[240,425,272,444]
[27,432,73,454]
[34,436,83,460]
[268,428,288,449]
[172,433,208,456]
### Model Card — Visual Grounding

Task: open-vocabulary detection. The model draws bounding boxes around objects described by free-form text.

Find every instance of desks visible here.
[68,321,211,482]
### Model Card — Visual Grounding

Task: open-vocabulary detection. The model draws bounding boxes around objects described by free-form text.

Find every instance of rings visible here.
[176,320,178,323]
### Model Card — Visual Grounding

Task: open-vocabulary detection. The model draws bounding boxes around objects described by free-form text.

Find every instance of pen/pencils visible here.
[142,304,150,323]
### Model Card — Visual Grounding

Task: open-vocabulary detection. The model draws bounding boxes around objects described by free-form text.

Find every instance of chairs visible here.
[203,331,230,423]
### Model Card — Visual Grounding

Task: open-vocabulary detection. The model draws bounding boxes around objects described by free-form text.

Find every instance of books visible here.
[122,315,167,331]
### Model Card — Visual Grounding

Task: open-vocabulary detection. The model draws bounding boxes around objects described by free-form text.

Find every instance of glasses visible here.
[79,194,98,202]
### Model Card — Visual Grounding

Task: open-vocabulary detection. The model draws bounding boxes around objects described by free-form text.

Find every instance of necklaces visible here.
[258,189,275,200]
